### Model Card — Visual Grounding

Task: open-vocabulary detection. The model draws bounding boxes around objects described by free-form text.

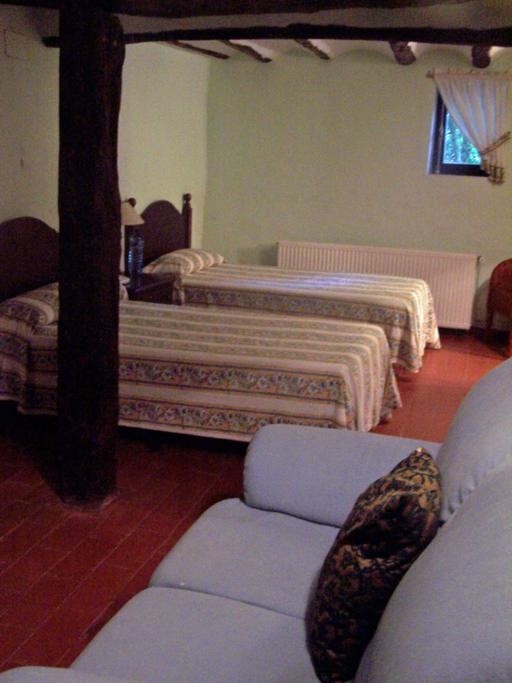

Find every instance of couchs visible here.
[1,357,512,683]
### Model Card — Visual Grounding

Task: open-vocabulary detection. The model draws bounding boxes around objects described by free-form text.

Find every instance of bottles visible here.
[128,230,144,287]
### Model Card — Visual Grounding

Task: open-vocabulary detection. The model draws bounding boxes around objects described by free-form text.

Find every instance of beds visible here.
[127,193,431,373]
[0,216,404,435]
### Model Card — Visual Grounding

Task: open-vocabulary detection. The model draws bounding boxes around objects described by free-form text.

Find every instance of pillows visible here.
[7,275,129,326]
[299,444,445,681]
[143,247,225,276]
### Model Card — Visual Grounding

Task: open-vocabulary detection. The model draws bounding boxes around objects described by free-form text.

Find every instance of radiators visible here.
[274,238,481,333]
